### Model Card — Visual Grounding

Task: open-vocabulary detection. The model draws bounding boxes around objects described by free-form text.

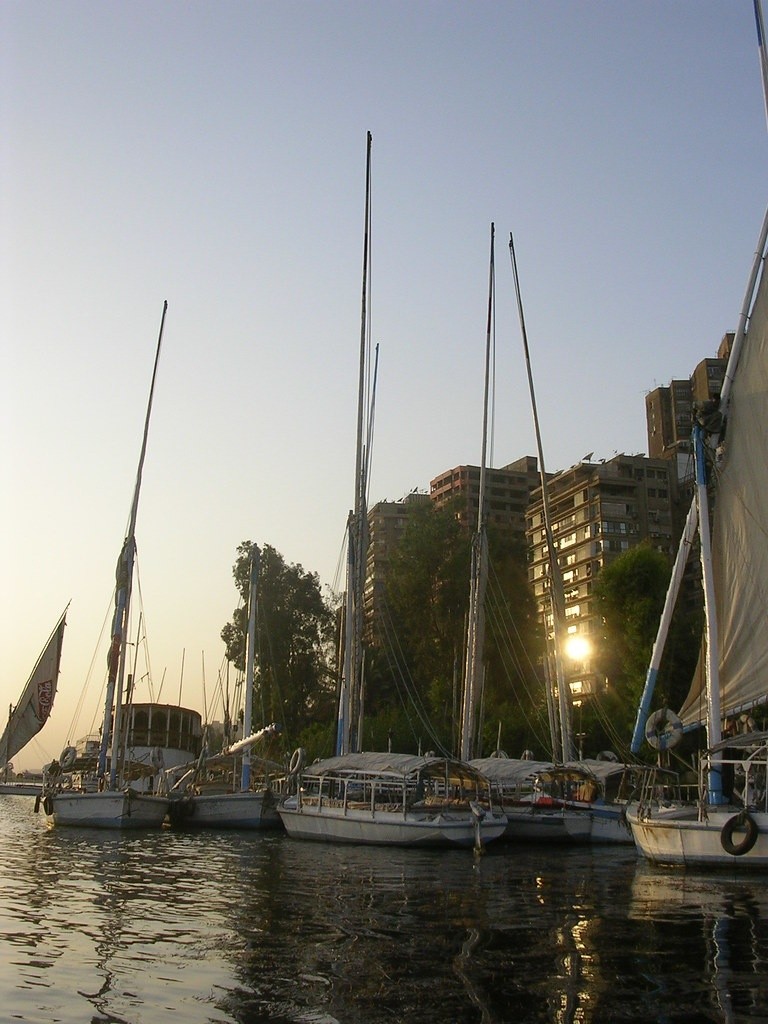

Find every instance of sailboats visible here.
[0,131,768,866]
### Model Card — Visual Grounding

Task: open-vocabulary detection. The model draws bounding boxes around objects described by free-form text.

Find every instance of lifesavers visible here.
[735,714,760,754]
[720,813,759,856]
[645,708,684,751]
[489,750,509,759]
[59,747,77,770]
[289,747,305,773]
[521,749,535,761]
[150,745,164,769]
[596,750,619,764]
